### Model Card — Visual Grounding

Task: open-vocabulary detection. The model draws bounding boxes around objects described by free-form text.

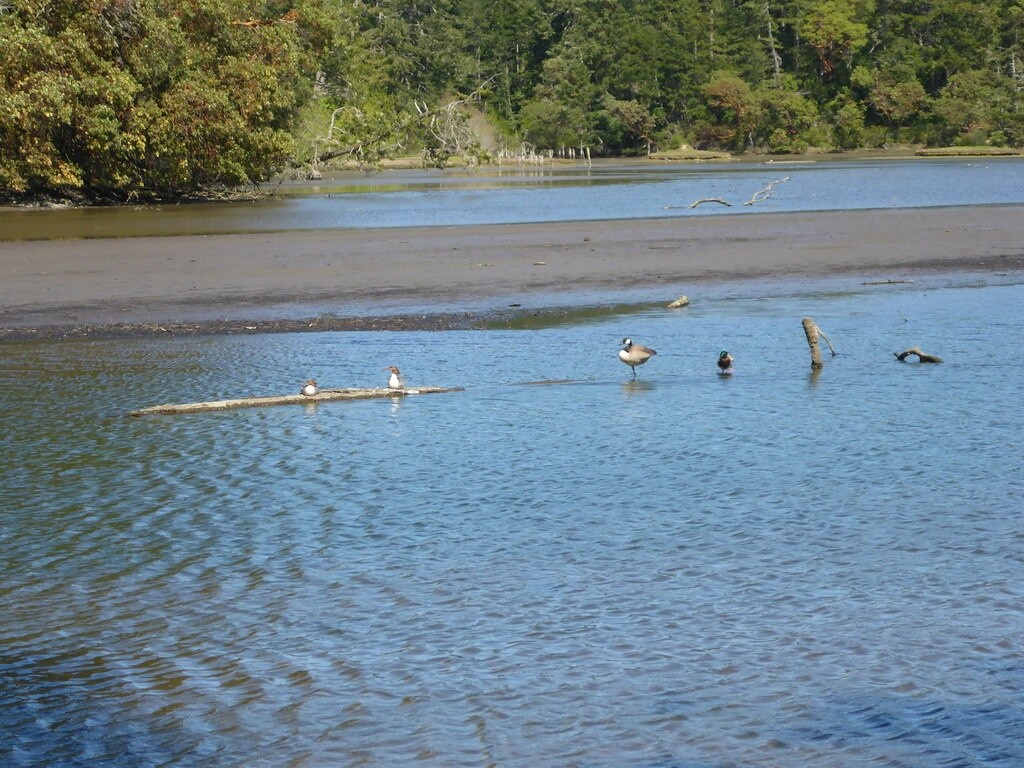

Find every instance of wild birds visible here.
[619,337,657,381]
[383,365,405,389]
[717,351,734,375]
[299,379,319,396]
